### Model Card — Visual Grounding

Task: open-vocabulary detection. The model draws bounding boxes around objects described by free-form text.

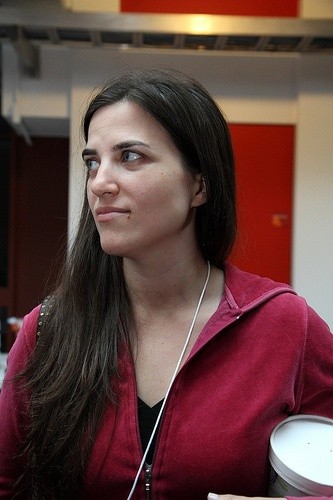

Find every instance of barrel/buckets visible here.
[269,414,333,499]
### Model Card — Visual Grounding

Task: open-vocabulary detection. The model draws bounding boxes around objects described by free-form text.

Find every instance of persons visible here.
[0,71,333,500]
